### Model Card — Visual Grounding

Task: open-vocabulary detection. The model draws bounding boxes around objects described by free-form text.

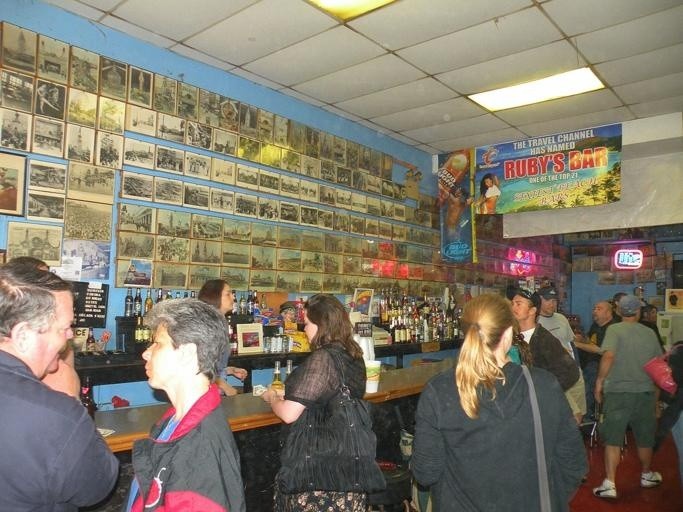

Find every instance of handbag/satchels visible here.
[642,344,683,394]
[277,349,387,494]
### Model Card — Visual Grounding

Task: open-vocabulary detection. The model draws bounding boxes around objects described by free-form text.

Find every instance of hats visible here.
[506,289,541,308]
[538,287,560,300]
[620,294,647,315]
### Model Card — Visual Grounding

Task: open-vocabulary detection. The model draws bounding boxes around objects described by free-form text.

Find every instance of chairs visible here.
[368,465,413,511]
[590,392,628,454]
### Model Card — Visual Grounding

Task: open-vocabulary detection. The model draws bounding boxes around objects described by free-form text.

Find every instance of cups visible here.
[352,334,376,363]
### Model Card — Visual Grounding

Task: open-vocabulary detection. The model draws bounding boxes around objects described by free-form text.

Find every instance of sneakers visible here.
[641,471,663,487]
[593,478,617,499]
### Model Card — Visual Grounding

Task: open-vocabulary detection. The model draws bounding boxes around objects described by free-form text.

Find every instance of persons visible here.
[0,263,118,510]
[440,183,471,248]
[192,279,248,397]
[264,295,373,511]
[594,294,661,500]
[654,339,683,454]
[477,174,499,213]
[535,289,587,432]
[513,288,578,391]
[569,301,615,430]
[128,298,247,511]
[605,286,666,327]
[410,295,589,511]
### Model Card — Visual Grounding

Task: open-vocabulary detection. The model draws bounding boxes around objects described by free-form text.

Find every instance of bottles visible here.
[410,326,416,343]
[276,335,283,352]
[176,292,180,297]
[145,289,153,312]
[144,325,151,342]
[80,378,95,418]
[232,290,238,315]
[393,321,400,343]
[253,290,259,315]
[166,290,172,300]
[271,337,276,352]
[418,302,464,340]
[184,292,189,298]
[246,290,253,314]
[190,291,195,298]
[380,289,420,327]
[400,320,406,344]
[240,295,246,314]
[135,316,144,342]
[156,288,163,303]
[264,337,272,353]
[272,361,283,391]
[86,328,95,355]
[134,288,141,316]
[125,288,133,317]
[260,294,267,310]
[289,337,293,352]
[282,335,289,352]
[286,361,293,378]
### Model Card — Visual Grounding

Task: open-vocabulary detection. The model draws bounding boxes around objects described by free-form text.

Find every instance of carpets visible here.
[568,417,683,511]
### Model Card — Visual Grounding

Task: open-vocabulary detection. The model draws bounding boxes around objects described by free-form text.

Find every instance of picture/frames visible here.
[299,273,323,293]
[655,269,665,283]
[359,144,371,173]
[651,255,673,269]
[392,224,407,242]
[234,192,258,218]
[257,196,280,222]
[300,154,321,179]
[209,187,235,215]
[336,189,351,210]
[407,245,423,263]
[212,128,237,157]
[0,68,35,115]
[382,152,393,181]
[435,282,448,296]
[1,249,6,264]
[27,190,66,223]
[422,281,434,297]
[188,264,221,290]
[638,257,651,270]
[156,112,186,145]
[363,257,379,277]
[26,157,68,194]
[124,103,157,137]
[395,279,409,294]
[395,243,408,262]
[117,202,156,234]
[638,244,657,257]
[219,95,240,135]
[324,253,344,275]
[434,266,447,282]
[343,237,362,256]
[277,248,301,271]
[191,214,223,241]
[119,170,154,202]
[370,149,383,178]
[423,264,433,281]
[95,130,124,171]
[63,198,112,242]
[0,21,38,77]
[352,170,368,192]
[656,282,667,295]
[116,231,155,261]
[409,280,422,296]
[31,116,65,158]
[378,278,395,295]
[154,261,190,289]
[318,208,334,230]
[236,163,259,191]
[394,181,406,202]
[377,242,394,260]
[124,137,156,170]
[435,248,441,266]
[251,223,278,248]
[587,246,604,257]
[256,108,274,145]
[355,322,373,337]
[395,261,408,279]
[251,245,277,270]
[6,221,63,267]
[335,165,352,187]
[343,254,363,276]
[423,247,435,265]
[665,289,683,313]
[236,323,264,354]
[64,122,96,163]
[616,271,634,284]
[301,229,325,253]
[394,202,406,222]
[319,184,336,206]
[344,275,362,294]
[574,246,587,255]
[153,176,183,206]
[221,266,250,292]
[183,181,210,211]
[96,95,126,136]
[249,270,276,292]
[447,267,456,283]
[408,262,423,281]
[153,72,178,116]
[334,136,347,167]
[456,269,465,282]
[281,148,301,174]
[301,250,324,273]
[36,33,70,86]
[636,270,655,283]
[381,178,394,199]
[455,283,465,294]
[319,130,334,164]
[379,260,395,278]
[66,160,115,205]
[273,114,289,150]
[351,193,367,213]
[127,64,154,110]
[423,230,432,246]
[199,88,220,129]
[380,200,394,219]
[288,119,306,155]
[260,142,281,168]
[351,288,374,318]
[72,280,110,328]
[62,240,110,280]
[465,269,473,283]
[155,234,190,264]
[156,208,192,238]
[433,232,441,247]
[237,135,261,164]
[378,220,392,240]
[321,159,336,183]
[177,81,200,123]
[322,274,344,293]
[278,227,302,250]
[222,242,251,268]
[190,239,222,266]
[239,102,260,142]
[591,256,611,272]
[572,256,591,272]
[33,77,68,122]
[477,214,571,302]
[65,86,98,130]
[325,234,344,254]
[366,197,380,217]
[406,227,423,245]
[367,174,381,194]
[365,217,379,237]
[222,219,252,245]
[417,192,433,212]
[648,296,664,307]
[598,271,616,285]
[405,206,432,228]
[346,140,359,170]
[300,179,319,203]
[186,120,213,151]
[115,260,154,288]
[279,200,300,224]
[184,151,212,181]
[360,277,377,295]
[362,239,377,258]
[99,55,128,103]
[69,45,101,94]
[212,158,236,186]
[0,108,32,152]
[304,125,321,158]
[300,205,319,228]
[334,211,349,232]
[349,214,365,235]
[280,175,300,199]
[155,144,184,175]
[0,151,28,217]
[276,272,300,293]
[604,246,621,257]
[258,168,281,195]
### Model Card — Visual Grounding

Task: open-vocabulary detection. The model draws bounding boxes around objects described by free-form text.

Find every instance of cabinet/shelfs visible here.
[78,339,460,412]
[90,385,425,512]
